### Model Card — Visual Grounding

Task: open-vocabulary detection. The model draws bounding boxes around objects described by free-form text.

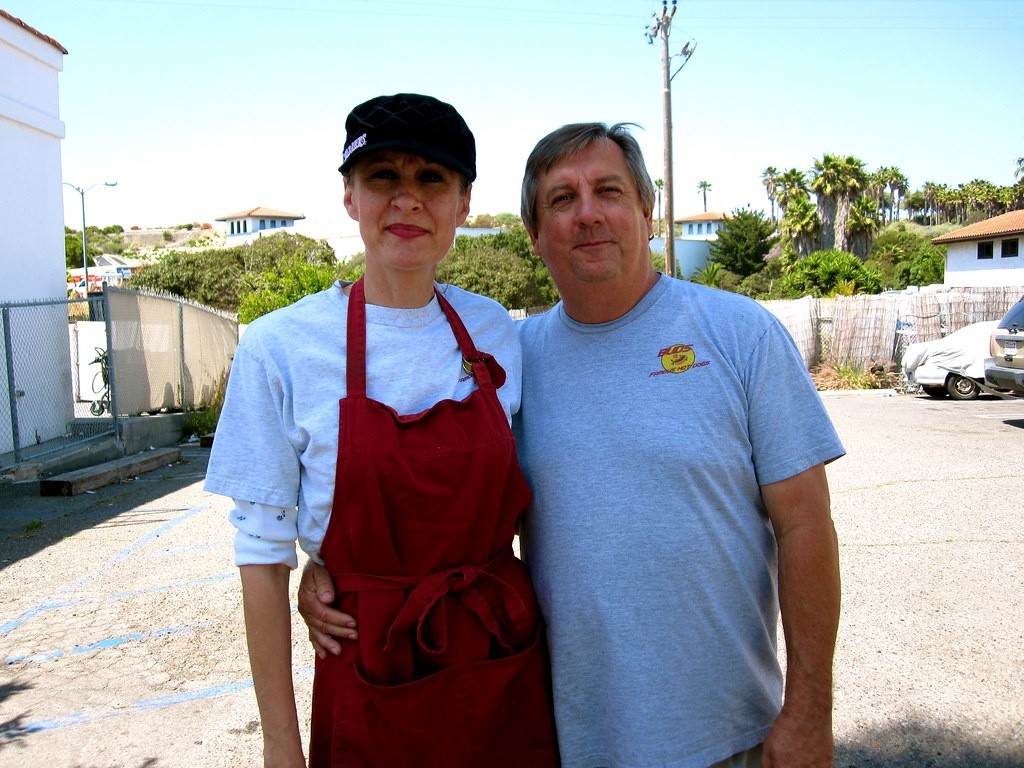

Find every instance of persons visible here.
[204,92,563,768]
[296,121,846,768]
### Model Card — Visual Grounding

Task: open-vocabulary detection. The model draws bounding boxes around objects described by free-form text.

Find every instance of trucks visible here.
[73,276,122,300]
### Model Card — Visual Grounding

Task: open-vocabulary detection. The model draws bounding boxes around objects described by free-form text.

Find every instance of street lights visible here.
[61,179,116,301]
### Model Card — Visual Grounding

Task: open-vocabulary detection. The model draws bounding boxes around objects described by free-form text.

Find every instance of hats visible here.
[338,93,477,178]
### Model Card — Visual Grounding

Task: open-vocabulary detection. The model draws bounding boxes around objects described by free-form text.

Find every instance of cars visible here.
[985,283,1024,398]
[903,320,1000,399]
